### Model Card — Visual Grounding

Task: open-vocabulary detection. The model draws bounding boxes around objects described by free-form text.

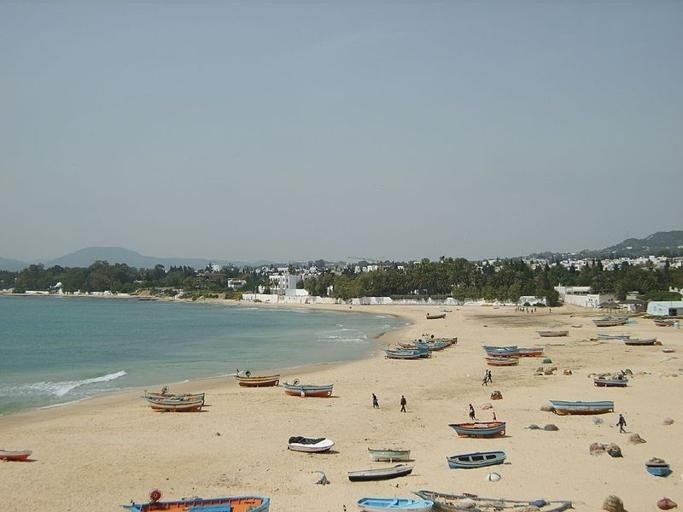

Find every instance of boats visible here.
[591,317,673,328]
[623,337,656,346]
[548,399,613,416]
[282,382,333,397]
[0,449,32,461]
[536,330,568,337]
[286,436,334,452]
[232,374,280,388]
[347,422,572,512]
[140,400,204,412]
[144,391,204,402]
[593,378,627,387]
[120,495,270,512]
[425,313,445,319]
[384,337,457,359]
[481,346,542,366]
[645,457,669,477]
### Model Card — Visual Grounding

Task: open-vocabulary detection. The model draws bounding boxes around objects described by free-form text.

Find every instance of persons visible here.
[487,370,492,383]
[371,392,380,408]
[481,369,488,386]
[400,394,407,412]
[616,413,627,433]
[468,403,476,419]
